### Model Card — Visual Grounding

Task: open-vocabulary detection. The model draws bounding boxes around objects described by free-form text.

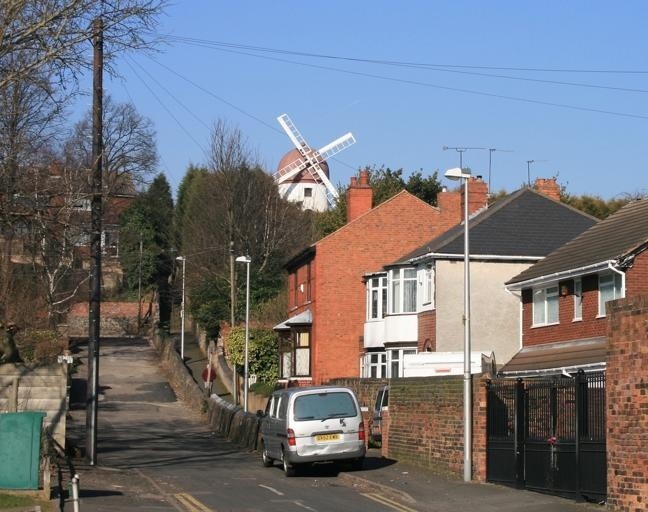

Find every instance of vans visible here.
[256,384,369,476]
[370,384,390,443]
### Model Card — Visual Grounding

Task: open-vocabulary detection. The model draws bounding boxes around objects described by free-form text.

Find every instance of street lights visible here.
[234,252,252,416]
[175,254,191,362]
[443,166,479,485]
